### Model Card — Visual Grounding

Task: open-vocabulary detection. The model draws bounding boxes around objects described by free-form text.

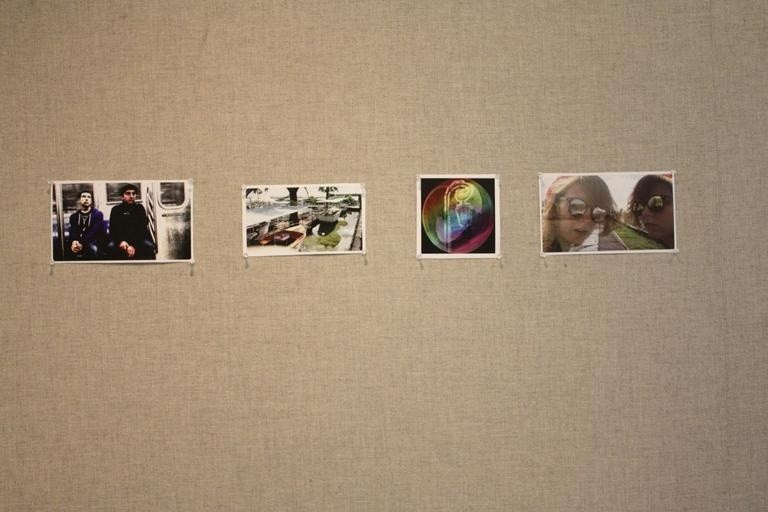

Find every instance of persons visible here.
[541,174,611,252]
[627,173,674,252]
[66,185,150,260]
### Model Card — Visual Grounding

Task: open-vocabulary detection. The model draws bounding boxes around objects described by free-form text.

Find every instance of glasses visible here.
[558,196,608,223]
[631,194,673,216]
